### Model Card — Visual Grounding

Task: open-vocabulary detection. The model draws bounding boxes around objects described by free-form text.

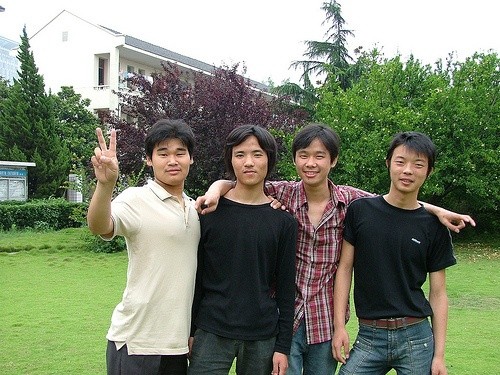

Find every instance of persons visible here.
[195,124,476,375]
[87,118,289,375]
[332,131,458,375]
[188,124,299,375]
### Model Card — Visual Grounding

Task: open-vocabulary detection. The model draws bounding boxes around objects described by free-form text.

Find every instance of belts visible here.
[359,317,426,330]
